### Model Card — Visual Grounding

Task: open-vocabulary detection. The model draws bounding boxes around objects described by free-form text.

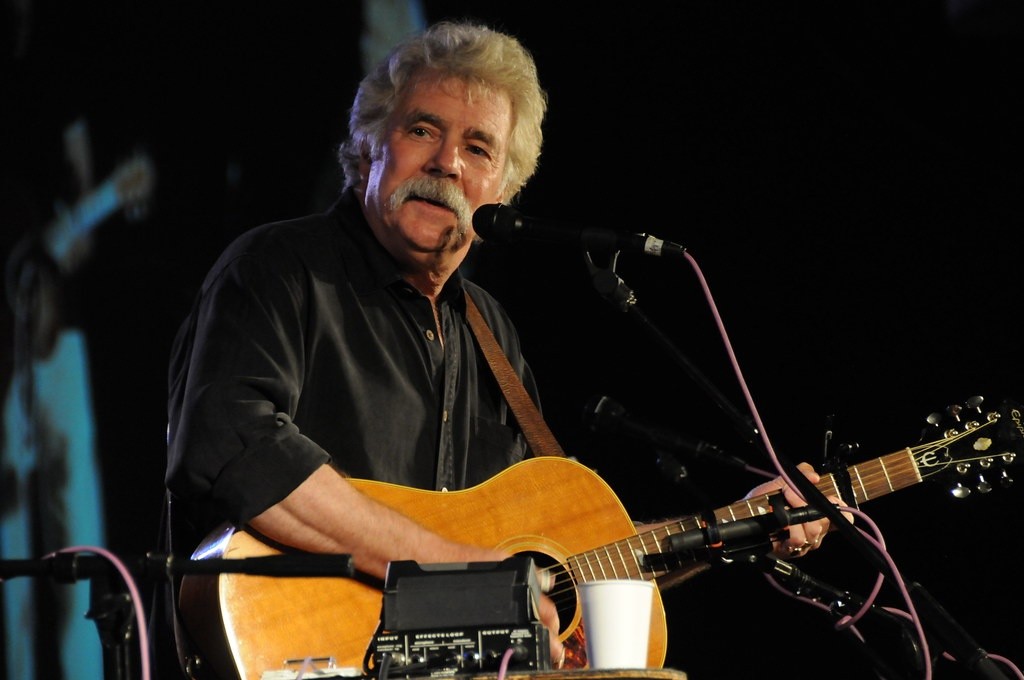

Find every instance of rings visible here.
[812,540,818,547]
[787,538,812,556]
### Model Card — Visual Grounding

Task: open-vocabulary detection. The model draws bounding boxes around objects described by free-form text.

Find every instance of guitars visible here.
[171,391,1023,679]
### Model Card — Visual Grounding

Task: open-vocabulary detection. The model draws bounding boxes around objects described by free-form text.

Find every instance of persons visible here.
[165,23,853,670]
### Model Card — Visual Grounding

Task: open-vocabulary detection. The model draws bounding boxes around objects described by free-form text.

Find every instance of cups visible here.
[577,580,655,670]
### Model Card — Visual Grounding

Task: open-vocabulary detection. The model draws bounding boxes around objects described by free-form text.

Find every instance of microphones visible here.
[579,394,780,485]
[470,202,689,259]
[667,504,823,552]
